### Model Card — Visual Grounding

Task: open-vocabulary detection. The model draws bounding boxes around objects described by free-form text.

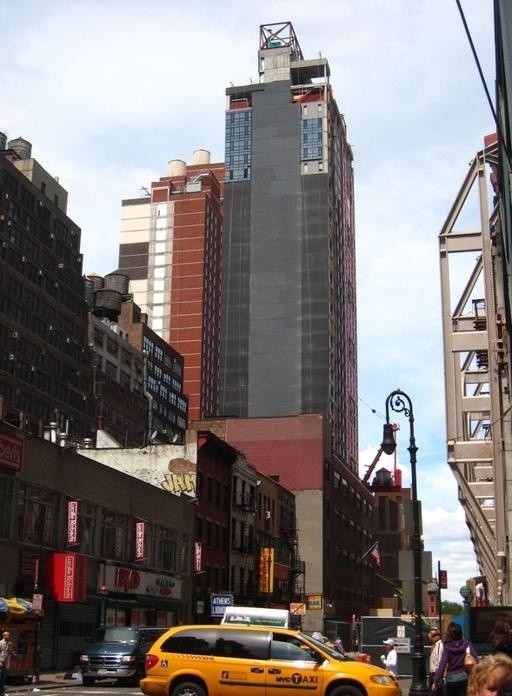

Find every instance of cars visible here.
[137,605,400,696]
[301,630,373,665]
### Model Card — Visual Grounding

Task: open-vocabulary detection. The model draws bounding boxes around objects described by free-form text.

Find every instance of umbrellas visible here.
[0,596,44,618]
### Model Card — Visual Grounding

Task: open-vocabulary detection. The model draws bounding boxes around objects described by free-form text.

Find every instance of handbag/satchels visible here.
[463,652,477,674]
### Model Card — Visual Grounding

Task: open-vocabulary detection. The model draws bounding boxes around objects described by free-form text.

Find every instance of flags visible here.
[371,542,382,567]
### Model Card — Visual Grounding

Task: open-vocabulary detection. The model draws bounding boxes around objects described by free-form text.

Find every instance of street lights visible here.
[381,388,428,696]
[97,583,111,627]
[422,578,443,639]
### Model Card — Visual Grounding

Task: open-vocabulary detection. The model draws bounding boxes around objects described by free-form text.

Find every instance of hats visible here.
[382,639,396,646]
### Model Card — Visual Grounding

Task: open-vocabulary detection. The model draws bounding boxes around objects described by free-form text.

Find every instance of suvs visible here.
[80,624,172,687]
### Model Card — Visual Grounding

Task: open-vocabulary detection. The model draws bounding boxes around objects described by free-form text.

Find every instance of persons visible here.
[334,635,342,648]
[380,638,398,679]
[305,631,324,644]
[292,641,309,659]
[0,631,12,696]
[428,619,512,696]
